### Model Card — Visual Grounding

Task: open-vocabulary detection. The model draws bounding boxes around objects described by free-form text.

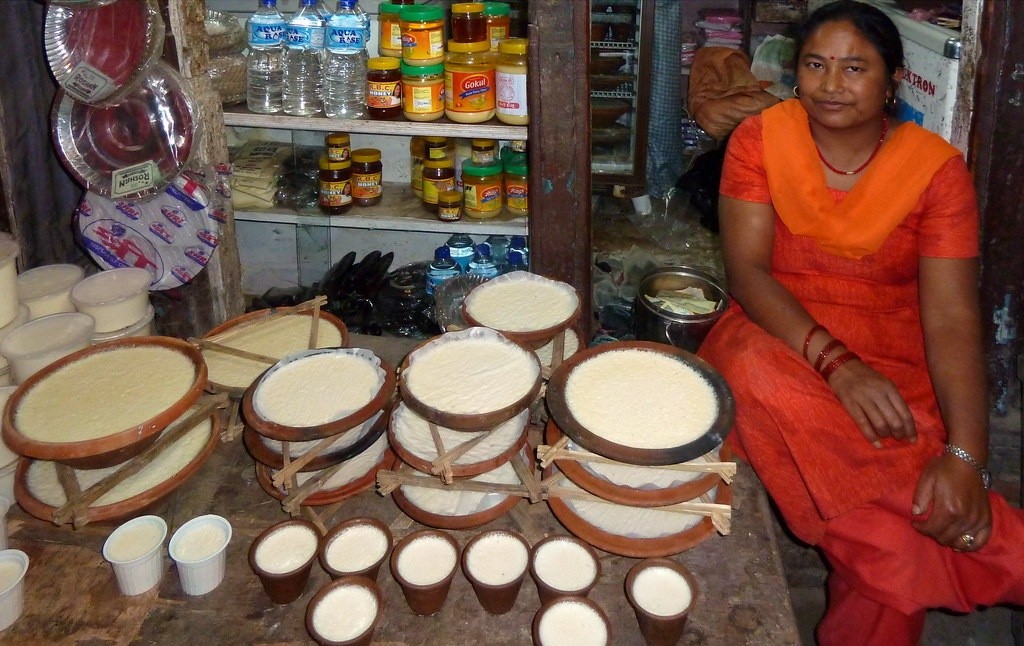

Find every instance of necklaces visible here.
[807,110,888,176]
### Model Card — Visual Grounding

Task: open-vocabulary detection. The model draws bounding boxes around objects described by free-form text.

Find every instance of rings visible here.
[961,534,974,547]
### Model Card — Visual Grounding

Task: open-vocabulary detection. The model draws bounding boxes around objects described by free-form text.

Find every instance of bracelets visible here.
[802,324,862,382]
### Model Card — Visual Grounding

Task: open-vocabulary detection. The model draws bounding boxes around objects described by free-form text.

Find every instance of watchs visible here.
[945,444,992,490]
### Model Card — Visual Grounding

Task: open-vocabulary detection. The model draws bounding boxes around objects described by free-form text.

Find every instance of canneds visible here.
[318,133,528,221]
[368,0,529,127]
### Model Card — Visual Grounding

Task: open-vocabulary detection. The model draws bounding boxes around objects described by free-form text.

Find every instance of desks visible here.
[0,397,801,646]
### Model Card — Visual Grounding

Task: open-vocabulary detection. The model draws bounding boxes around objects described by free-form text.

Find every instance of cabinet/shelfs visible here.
[169,0,594,345]
[589,0,655,207]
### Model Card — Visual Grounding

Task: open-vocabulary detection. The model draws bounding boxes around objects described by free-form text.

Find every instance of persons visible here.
[700,0,1024,646]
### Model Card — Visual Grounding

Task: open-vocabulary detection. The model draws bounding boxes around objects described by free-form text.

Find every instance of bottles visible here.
[424,233,531,303]
[244,0,372,119]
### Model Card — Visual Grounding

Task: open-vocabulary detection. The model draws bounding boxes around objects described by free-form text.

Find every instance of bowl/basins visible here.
[0,380,23,510]
[591,98,631,125]
[590,75,636,88]
[0,232,157,385]
[591,56,627,73]
[630,268,730,355]
[590,22,608,41]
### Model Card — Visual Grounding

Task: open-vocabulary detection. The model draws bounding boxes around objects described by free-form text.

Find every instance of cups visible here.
[102,515,168,596]
[530,595,613,646]
[611,23,631,43]
[305,575,385,645]
[460,529,532,615]
[168,516,233,595]
[528,533,602,605]
[248,519,323,605]
[318,516,394,582]
[389,528,462,615]
[624,556,700,645]
[0,547,29,631]
[0,497,11,550]
